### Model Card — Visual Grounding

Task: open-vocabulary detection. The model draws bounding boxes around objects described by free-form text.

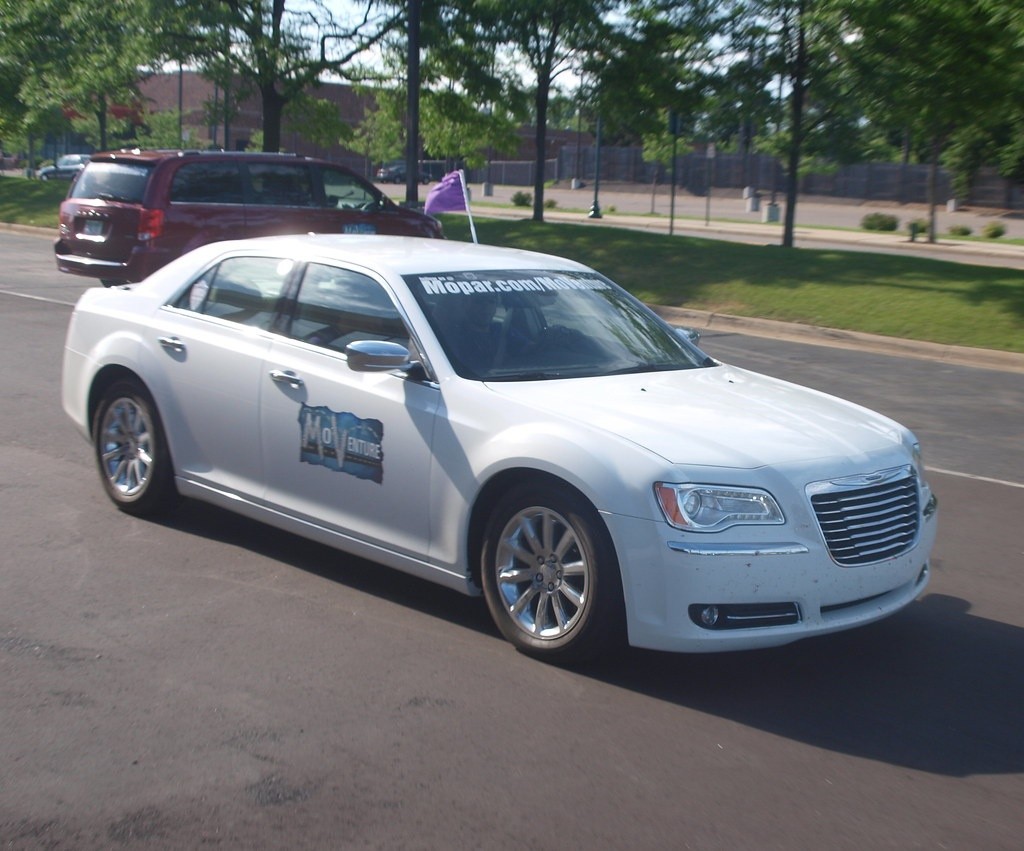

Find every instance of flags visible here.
[420,171,469,219]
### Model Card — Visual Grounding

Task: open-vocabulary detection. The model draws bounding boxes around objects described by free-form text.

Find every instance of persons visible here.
[452,282,566,376]
[303,288,394,365]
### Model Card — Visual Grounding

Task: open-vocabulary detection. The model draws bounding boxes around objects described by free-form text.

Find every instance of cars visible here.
[61,232,935,658]
[36,153,96,181]
[57,148,447,290]
